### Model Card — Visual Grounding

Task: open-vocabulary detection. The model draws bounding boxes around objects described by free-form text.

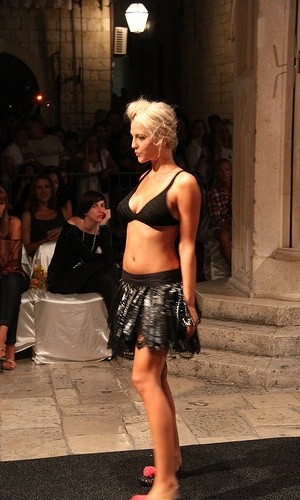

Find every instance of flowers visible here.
[30,263,48,289]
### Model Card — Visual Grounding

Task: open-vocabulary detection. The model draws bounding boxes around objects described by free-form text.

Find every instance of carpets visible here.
[1,437,300,500]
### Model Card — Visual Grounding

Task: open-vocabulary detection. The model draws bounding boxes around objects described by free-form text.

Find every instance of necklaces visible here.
[81,218,99,253]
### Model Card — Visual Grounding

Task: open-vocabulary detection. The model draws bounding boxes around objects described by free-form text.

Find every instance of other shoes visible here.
[0,344,6,361]
[3,358,16,369]
[137,463,184,486]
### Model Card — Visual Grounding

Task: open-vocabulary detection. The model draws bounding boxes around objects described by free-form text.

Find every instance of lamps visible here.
[125,2,155,39]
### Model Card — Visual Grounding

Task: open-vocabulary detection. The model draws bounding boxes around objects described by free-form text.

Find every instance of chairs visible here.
[15,242,112,364]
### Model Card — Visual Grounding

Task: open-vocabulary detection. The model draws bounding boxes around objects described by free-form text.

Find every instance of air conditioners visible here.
[114,27,128,54]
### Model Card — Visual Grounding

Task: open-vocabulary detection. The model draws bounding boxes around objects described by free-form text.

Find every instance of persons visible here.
[0,88,236,370]
[107,98,202,500]
